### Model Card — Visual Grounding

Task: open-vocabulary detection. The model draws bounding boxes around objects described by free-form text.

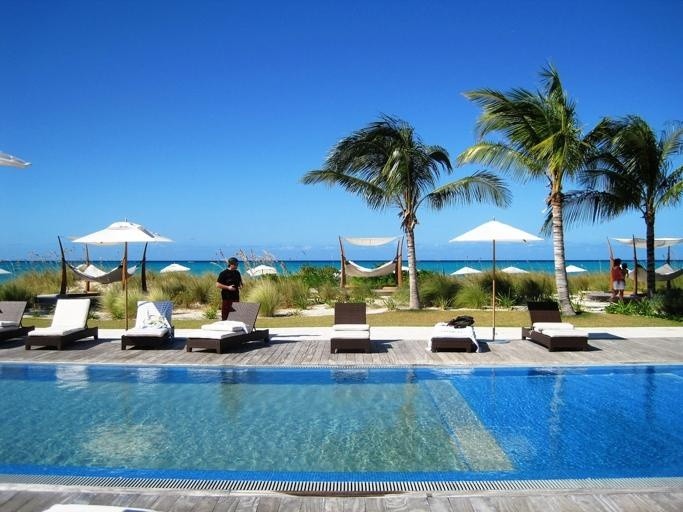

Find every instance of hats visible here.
[228,258,239,265]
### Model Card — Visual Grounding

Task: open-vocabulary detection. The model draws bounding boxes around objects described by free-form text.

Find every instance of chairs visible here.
[521,301,589,352]
[184,301,269,354]
[426,318,480,352]
[25,298,98,350]
[330,302,370,354]
[121,301,174,350]
[0,301,35,348]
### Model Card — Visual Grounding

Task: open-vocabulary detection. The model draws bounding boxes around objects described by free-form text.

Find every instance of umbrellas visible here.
[621,263,629,278]
[448,216,545,337]
[216,257,244,321]
[71,218,177,331]
[246,263,278,277]
[611,258,625,303]
[159,262,191,274]
[500,265,530,273]
[450,266,482,276]
[566,264,588,273]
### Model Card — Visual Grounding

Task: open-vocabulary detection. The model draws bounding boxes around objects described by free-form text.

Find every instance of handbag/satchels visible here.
[448,315,474,328]
